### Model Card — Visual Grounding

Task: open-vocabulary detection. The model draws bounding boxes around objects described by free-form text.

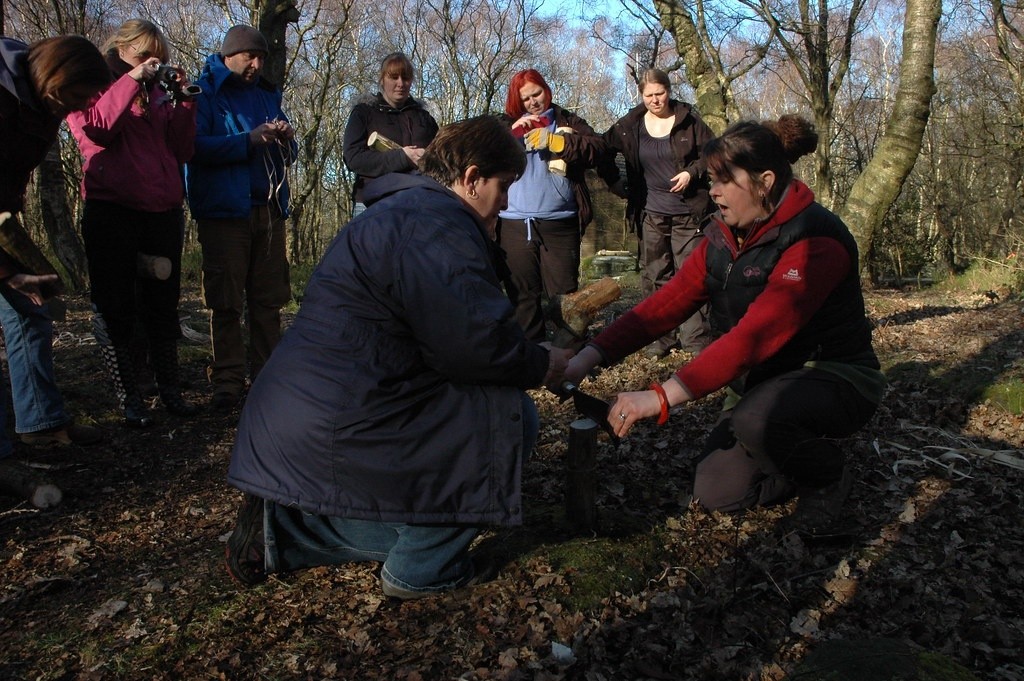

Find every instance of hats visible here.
[221,25,269,59]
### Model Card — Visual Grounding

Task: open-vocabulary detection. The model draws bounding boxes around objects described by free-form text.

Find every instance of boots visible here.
[104,346,198,428]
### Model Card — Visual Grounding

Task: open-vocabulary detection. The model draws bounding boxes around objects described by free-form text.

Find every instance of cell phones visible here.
[513,116,550,138]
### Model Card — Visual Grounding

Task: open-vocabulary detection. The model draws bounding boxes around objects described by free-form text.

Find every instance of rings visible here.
[618,413,626,421]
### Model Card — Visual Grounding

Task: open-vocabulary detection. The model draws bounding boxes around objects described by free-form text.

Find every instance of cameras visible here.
[155,64,179,81]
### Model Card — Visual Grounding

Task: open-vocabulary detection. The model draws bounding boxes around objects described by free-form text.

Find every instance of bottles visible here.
[549,120,576,176]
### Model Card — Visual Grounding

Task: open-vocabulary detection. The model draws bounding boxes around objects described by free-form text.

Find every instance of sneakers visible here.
[227,492,269,588]
[382,548,497,596]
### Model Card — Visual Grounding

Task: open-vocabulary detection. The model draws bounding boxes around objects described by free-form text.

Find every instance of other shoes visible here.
[21,422,101,446]
[648,338,680,359]
[796,468,854,525]
[213,389,242,410]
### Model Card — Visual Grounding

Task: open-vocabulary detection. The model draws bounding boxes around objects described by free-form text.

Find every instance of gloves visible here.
[524,128,564,153]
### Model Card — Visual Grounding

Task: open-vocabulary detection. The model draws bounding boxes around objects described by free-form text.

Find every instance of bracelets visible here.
[649,384,668,424]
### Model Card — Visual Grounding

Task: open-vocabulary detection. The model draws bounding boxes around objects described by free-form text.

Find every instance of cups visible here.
[367,131,402,152]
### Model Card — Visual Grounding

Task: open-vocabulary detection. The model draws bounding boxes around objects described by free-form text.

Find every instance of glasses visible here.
[130,45,160,64]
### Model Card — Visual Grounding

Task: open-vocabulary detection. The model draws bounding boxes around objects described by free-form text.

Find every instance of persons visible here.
[66,20,202,424]
[548,114,887,532]
[0,36,110,450]
[494,73,602,345]
[597,69,715,361]
[226,114,571,597]
[342,52,438,220]
[184,26,297,407]
